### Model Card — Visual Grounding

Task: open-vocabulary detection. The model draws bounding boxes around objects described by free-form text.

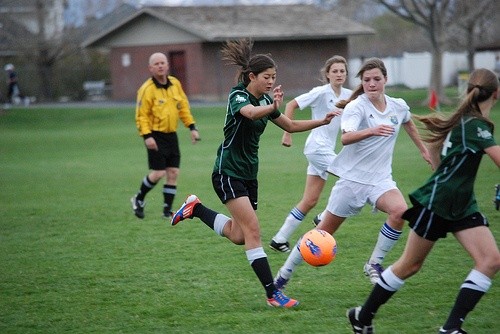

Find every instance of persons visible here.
[347,67,500,334]
[272,58,434,290]
[129,53,200,219]
[269,55,353,253]
[495,184,500,210]
[3,63,30,107]
[171,38,340,310]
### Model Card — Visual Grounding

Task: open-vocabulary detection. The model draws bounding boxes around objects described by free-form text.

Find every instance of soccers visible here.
[300,228,337,267]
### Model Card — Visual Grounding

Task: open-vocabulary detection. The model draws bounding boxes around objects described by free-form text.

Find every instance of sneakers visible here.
[266,291,299,309]
[439,324,468,334]
[312,212,322,228]
[268,236,292,254]
[346,306,373,334]
[275,270,290,292]
[130,193,145,219]
[169,193,202,226]
[363,259,384,285]
[160,210,177,220]
